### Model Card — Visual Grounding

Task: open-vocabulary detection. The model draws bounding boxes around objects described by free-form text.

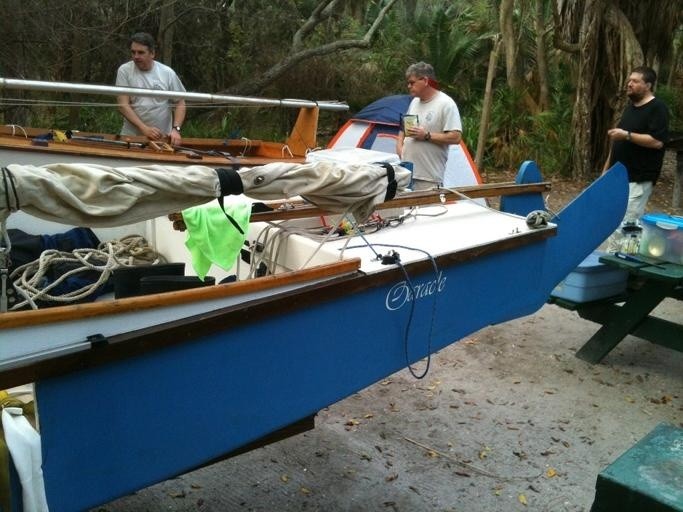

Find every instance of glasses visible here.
[407,77,423,85]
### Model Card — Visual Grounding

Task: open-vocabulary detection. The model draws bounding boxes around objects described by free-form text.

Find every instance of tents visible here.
[324,93,488,208]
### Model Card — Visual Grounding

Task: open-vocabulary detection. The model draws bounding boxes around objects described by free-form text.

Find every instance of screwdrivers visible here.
[615,251,667,270]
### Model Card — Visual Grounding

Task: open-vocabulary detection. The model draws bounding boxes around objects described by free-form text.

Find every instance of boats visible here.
[0,77,631,511]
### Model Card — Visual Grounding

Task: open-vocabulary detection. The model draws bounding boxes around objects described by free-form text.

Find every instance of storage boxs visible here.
[640,213,683,263]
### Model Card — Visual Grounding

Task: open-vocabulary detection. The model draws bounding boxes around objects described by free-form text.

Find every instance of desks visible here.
[575,252,683,364]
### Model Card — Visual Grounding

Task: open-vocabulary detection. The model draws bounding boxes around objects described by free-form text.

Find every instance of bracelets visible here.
[625,130,630,141]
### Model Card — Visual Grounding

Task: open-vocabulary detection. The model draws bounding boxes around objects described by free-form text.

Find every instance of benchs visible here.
[545,295,629,310]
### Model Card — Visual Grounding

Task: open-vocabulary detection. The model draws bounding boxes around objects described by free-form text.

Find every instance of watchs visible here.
[423,132,430,142]
[171,125,181,132]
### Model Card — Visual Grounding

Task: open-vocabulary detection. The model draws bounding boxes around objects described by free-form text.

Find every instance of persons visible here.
[598,66,669,254]
[394,60,462,191]
[113,31,188,150]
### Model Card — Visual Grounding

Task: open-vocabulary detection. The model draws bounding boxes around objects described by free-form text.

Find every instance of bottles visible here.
[628,235,638,255]
